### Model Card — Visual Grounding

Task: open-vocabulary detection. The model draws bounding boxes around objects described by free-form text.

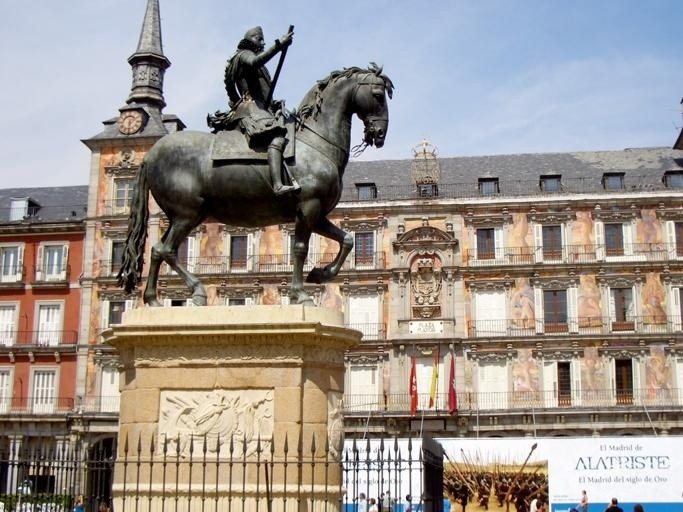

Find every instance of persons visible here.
[632,502,643,511]
[605,497,623,511]
[97,501,111,512]
[443,465,550,512]
[73,494,85,512]
[578,487,588,512]
[352,488,414,512]
[223,24,297,196]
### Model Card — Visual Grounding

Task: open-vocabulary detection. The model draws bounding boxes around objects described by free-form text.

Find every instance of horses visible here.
[116,60,396,308]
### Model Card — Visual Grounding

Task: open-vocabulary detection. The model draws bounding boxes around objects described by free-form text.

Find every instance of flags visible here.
[429,347,439,413]
[408,355,418,418]
[447,352,457,415]
